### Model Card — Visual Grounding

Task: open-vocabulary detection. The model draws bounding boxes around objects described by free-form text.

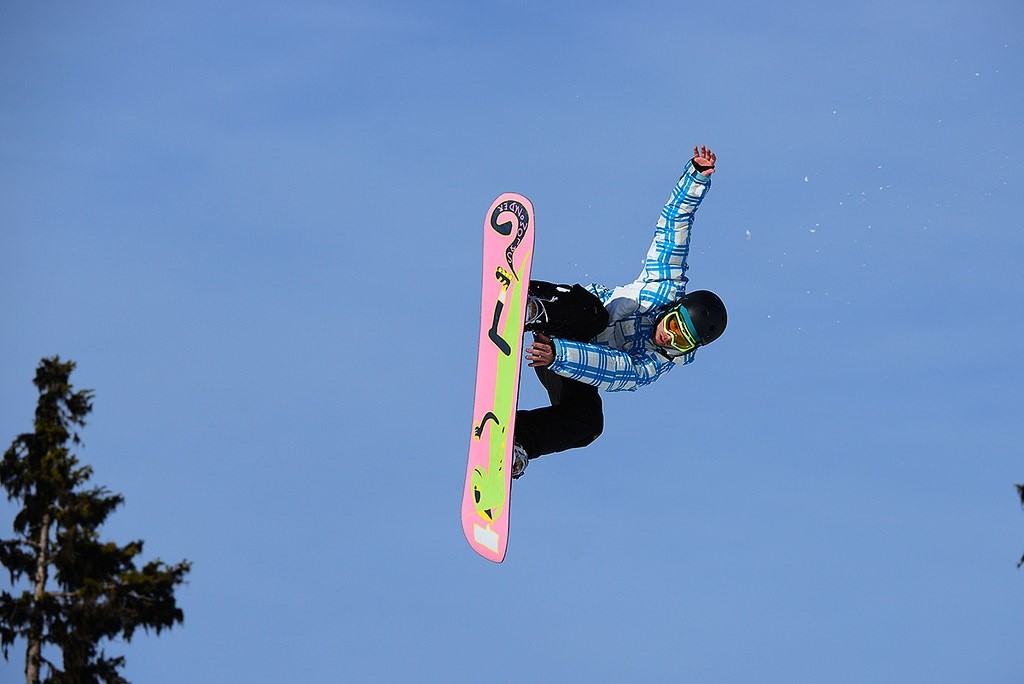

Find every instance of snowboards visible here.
[461,193,536,564]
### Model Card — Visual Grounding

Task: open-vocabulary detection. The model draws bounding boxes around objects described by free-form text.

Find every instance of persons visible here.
[511,143,727,481]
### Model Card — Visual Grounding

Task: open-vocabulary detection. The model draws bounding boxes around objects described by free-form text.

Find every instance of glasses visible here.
[663,311,695,353]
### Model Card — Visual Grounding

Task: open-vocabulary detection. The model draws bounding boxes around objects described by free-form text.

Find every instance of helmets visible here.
[677,290,728,346]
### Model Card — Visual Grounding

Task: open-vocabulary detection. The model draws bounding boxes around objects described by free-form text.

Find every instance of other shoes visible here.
[526,299,536,323]
[512,442,528,476]
